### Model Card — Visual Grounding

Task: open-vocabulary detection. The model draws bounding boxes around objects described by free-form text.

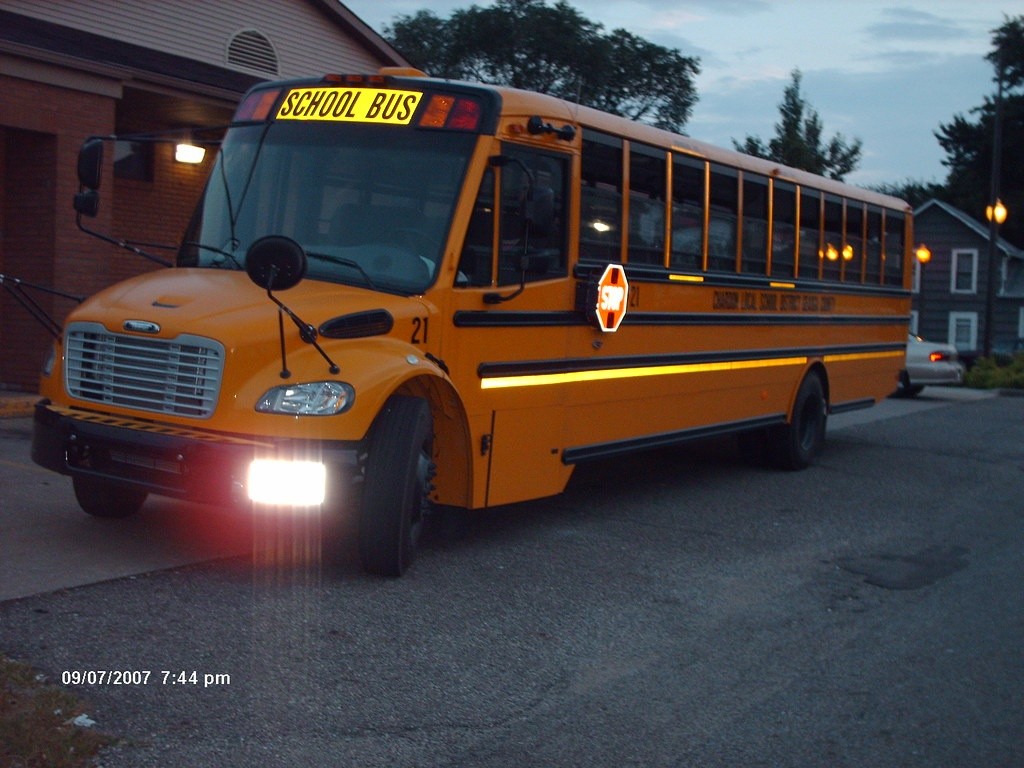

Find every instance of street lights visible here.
[977,101,1009,364]
[914,243,933,336]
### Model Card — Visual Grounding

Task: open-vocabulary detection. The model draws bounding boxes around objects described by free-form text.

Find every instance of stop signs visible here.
[593,264,629,333]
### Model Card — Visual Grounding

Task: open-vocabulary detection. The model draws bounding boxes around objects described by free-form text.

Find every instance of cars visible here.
[886,326,964,398]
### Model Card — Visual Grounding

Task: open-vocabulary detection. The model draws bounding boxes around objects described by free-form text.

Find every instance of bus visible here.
[0,65,916,578]
